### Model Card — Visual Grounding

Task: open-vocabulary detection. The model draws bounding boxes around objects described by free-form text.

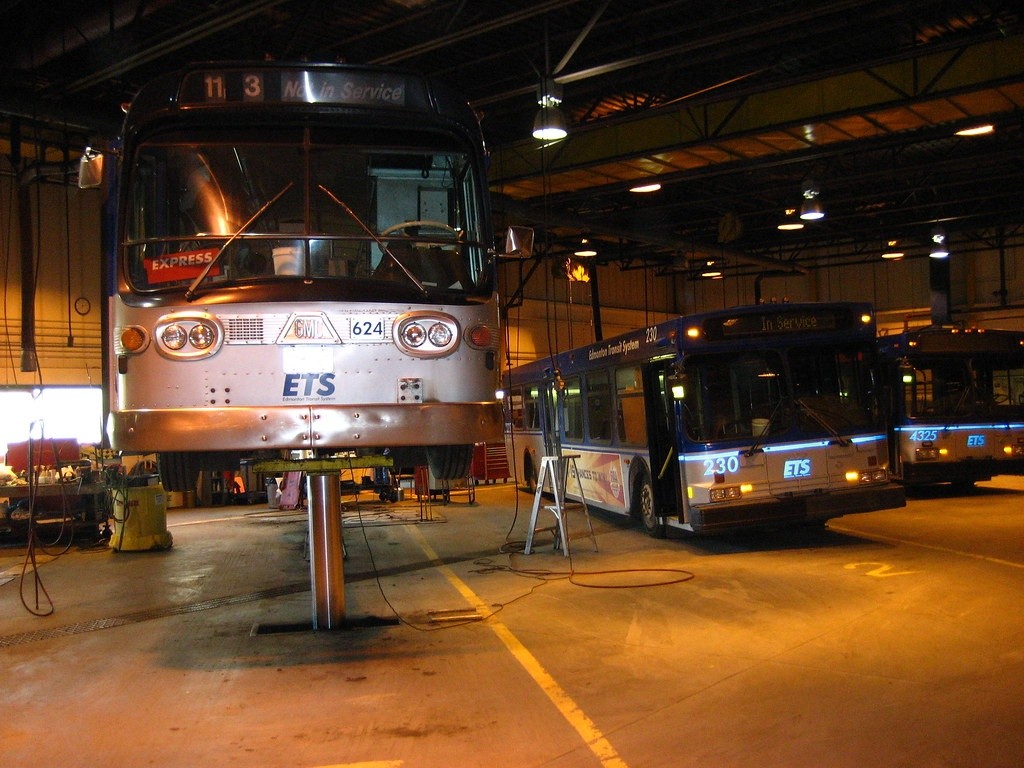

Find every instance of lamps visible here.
[701,257,721,277]
[573,234,598,257]
[928,241,950,260]
[532,82,568,140]
[799,180,825,220]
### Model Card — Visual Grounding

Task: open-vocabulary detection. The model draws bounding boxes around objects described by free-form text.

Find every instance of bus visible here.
[850,319,1024,498]
[502,301,907,540]
[73,50,537,493]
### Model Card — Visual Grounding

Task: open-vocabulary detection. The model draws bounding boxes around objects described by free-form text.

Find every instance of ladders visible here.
[523,454,598,557]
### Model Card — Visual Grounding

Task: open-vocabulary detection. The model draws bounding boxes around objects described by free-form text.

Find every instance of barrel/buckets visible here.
[265,477,282,509]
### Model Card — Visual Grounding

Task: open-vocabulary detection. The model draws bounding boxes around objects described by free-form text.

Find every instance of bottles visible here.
[32,465,59,484]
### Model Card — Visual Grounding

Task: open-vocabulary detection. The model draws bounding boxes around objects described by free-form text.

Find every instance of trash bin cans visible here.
[265,476,284,509]
